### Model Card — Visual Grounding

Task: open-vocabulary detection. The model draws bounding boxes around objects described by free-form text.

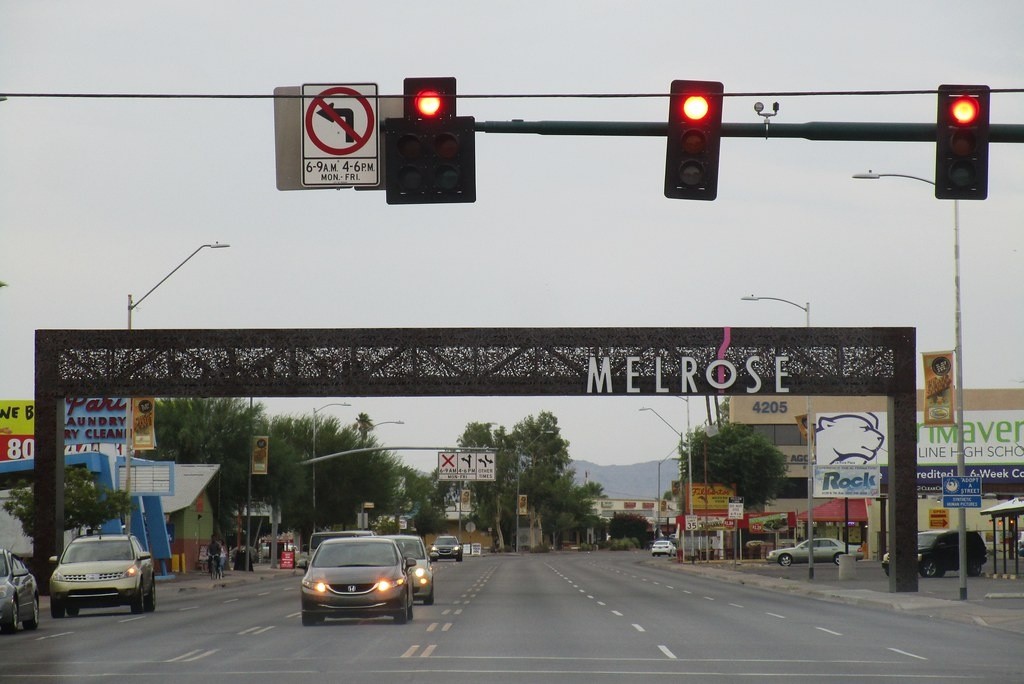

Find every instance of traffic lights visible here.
[664,80,723,200]
[937,84,991,200]
[384,78,477,204]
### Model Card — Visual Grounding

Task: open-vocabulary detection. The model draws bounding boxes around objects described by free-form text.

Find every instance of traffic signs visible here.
[438,452,496,481]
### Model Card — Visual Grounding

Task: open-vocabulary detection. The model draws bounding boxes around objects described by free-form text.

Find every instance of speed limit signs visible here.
[685,515,698,531]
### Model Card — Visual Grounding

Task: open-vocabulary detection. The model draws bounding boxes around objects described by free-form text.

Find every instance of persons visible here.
[217,539,228,577]
[206,535,221,573]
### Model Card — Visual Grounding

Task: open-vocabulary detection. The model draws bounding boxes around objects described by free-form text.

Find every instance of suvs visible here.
[881,529,988,579]
[48,533,156,618]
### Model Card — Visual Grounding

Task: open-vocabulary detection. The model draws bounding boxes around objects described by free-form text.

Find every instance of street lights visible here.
[852,174,968,603]
[639,408,683,491]
[125,244,230,534]
[741,296,814,578]
[313,403,351,533]
[516,430,554,553]
[361,421,405,448]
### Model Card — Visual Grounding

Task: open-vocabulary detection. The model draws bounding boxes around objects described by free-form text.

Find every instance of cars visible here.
[766,538,864,567]
[0,549,41,634]
[300,530,439,626]
[430,536,464,562]
[652,534,679,557]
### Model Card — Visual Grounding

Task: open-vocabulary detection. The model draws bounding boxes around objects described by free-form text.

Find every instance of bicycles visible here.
[208,554,223,580]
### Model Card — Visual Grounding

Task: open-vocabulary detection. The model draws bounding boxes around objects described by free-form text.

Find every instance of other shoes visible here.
[208,570,211,573]
[222,573,224,577]
[216,569,219,573]
